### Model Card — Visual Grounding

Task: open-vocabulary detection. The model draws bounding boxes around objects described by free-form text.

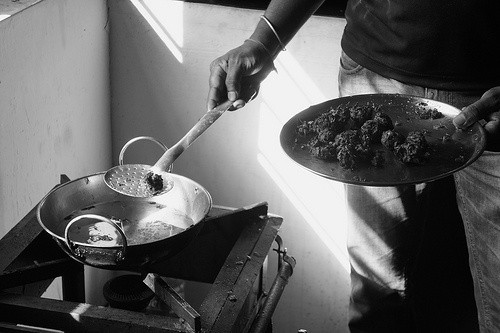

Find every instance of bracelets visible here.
[261,15,286,52]
[245,39,278,74]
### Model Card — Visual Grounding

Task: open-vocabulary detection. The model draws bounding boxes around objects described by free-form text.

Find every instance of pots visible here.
[36,136,214,269]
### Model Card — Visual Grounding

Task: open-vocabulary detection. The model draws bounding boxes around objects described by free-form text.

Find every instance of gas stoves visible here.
[1,169,297,333]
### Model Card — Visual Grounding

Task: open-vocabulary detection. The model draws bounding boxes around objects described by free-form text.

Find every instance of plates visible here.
[278,94,487,187]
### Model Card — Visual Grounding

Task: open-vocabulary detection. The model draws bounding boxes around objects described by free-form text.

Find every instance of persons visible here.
[208,0,500,333]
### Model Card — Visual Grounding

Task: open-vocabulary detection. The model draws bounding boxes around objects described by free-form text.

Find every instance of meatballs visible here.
[298,102,429,171]
[144,172,164,192]
